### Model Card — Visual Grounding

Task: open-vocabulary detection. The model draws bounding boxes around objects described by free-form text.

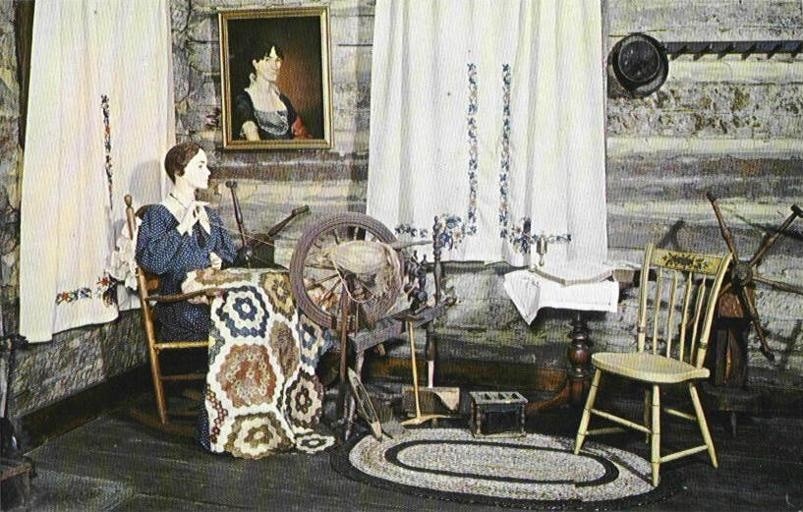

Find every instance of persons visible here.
[133,140,239,456]
[233,31,313,140]
